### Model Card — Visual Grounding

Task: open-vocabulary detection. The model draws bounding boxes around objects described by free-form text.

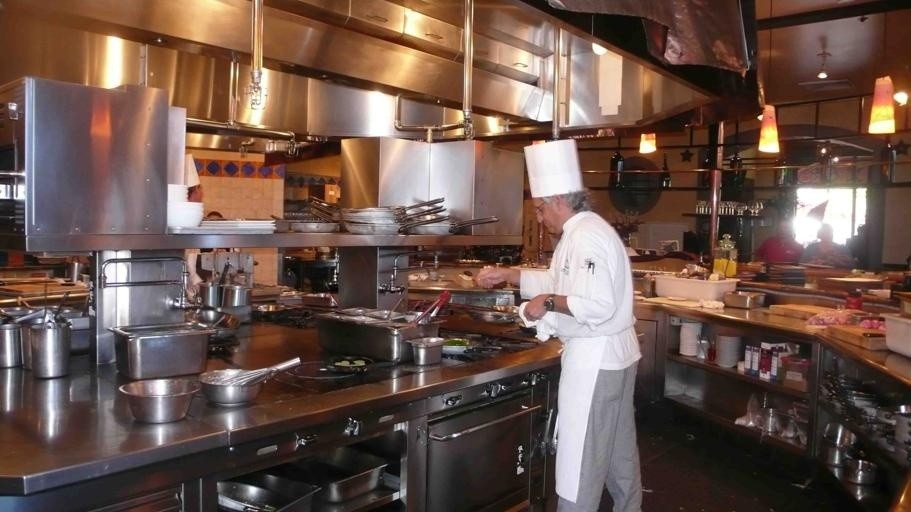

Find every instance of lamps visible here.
[638,132,658,156]
[816,52,832,79]
[867,0,897,136]
[758,0,782,155]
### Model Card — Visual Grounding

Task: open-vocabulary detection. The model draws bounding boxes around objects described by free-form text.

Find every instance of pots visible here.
[469,306,520,325]
[272,195,500,233]
[196,261,252,307]
[821,435,879,485]
[325,354,396,376]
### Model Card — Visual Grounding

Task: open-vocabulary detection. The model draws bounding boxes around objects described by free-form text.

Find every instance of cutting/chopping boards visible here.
[825,324,890,353]
[769,303,837,319]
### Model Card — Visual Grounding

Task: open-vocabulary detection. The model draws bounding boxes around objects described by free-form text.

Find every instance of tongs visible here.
[410,289,451,329]
[385,295,409,321]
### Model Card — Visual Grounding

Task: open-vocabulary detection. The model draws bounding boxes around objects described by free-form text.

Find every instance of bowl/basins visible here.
[200,369,266,407]
[257,303,286,317]
[119,376,200,423]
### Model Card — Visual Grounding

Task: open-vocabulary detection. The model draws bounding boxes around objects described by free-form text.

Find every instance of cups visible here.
[0,317,72,378]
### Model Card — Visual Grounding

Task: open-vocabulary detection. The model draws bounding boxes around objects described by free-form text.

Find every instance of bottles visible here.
[697,333,716,361]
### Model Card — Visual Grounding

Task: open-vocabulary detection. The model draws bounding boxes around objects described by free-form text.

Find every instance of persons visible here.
[196,208,233,283]
[471,186,645,511]
[181,181,206,304]
[751,218,873,266]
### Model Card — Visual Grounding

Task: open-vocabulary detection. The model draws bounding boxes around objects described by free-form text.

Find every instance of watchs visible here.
[543,294,555,312]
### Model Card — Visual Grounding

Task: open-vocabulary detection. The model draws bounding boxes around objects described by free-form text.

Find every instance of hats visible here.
[524,139,584,199]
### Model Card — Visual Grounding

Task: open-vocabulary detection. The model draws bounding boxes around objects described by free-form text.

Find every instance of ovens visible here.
[425,382,550,511]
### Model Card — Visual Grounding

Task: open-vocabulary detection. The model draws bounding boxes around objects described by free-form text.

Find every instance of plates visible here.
[678,320,705,356]
[164,183,277,236]
[715,333,744,369]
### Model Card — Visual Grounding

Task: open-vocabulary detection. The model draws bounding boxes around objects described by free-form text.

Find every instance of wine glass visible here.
[692,199,763,215]
